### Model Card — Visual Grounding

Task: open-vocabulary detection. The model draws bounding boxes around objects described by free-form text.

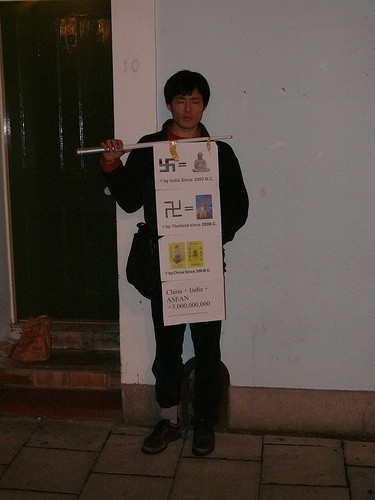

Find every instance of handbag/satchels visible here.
[126,223,161,300]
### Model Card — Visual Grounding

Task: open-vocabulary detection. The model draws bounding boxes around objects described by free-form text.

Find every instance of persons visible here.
[100,70,250,456]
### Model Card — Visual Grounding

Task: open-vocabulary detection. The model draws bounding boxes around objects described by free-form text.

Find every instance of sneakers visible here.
[141,417,182,455]
[192,422,215,456]
